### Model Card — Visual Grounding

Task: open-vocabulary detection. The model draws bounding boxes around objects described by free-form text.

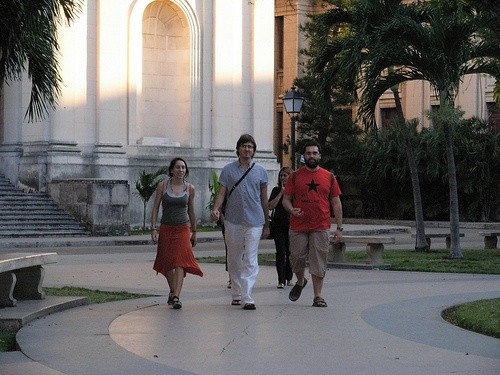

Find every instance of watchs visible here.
[337,226,344,231]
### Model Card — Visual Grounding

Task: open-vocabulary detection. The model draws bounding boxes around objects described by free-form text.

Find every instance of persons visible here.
[216,198,231,288]
[150,157,203,308]
[282,140,344,307]
[212,134,271,310]
[268,167,294,289]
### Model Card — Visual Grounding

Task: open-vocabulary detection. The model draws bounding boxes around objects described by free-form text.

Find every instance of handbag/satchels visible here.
[266,216,275,239]
[217,199,227,228]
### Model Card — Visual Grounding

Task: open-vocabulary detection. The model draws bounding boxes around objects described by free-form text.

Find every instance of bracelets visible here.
[192,230,197,232]
[151,224,157,230]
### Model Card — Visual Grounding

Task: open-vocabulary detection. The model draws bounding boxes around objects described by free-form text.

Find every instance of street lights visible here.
[281,85,304,174]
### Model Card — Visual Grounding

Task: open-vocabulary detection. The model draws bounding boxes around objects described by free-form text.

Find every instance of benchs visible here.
[0,252,58,308]
[479,232,500,249]
[329,235,396,265]
[411,233,465,248]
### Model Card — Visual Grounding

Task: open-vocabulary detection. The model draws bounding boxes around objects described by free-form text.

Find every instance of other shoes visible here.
[227,281,231,288]
[277,283,284,288]
[287,280,293,286]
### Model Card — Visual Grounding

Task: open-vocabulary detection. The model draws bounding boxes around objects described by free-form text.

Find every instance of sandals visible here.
[243,303,256,309]
[168,291,174,305]
[172,296,182,309]
[232,300,242,305]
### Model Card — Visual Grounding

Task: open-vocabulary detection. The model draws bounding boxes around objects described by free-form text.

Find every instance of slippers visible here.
[313,296,327,307]
[289,278,307,301]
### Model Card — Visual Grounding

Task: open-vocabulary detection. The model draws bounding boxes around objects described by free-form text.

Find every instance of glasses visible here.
[238,145,254,148]
